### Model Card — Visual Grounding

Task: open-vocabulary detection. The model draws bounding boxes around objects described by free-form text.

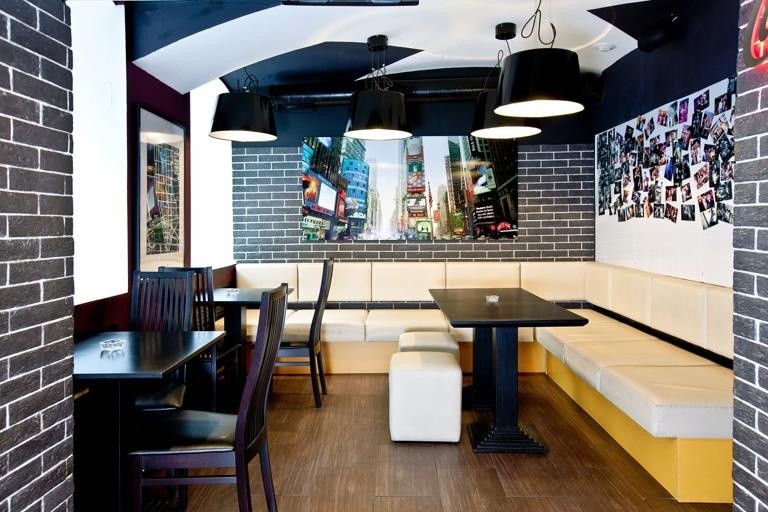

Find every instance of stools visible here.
[388,352,462,444]
[399,331,460,362]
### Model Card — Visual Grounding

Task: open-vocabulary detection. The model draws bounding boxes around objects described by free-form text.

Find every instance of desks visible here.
[194,288,294,403]
[428,289,588,453]
[74,331,226,512]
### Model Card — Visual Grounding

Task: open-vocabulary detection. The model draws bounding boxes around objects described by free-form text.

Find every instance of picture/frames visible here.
[134,103,186,282]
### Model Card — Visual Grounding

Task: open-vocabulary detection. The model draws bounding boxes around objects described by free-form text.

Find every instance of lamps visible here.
[467,51,541,141]
[343,37,411,140]
[483,23,584,117]
[208,74,278,145]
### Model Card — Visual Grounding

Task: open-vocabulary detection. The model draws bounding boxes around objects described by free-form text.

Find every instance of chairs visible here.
[159,267,242,389]
[128,271,192,411]
[249,256,334,408]
[128,284,288,511]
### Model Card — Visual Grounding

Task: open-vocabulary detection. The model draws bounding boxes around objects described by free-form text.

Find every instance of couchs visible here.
[214,262,733,502]
